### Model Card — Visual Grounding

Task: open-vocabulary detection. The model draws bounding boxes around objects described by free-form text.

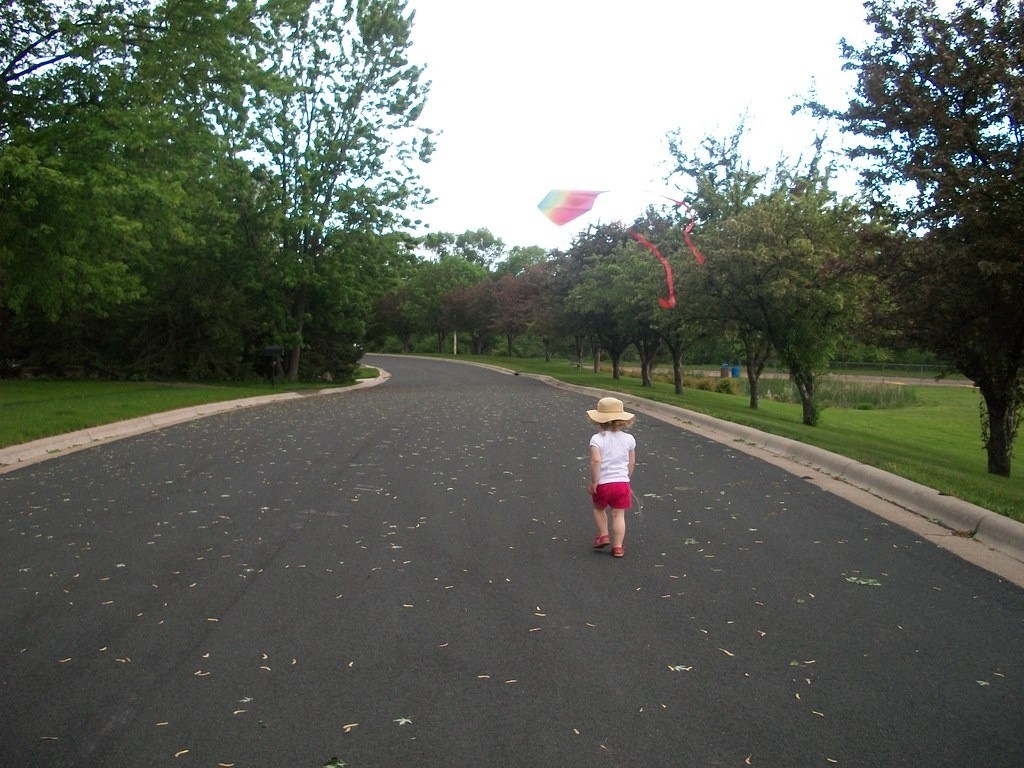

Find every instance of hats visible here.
[587,397,635,423]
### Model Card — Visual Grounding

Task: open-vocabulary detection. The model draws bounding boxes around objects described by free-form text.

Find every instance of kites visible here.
[536,188,703,309]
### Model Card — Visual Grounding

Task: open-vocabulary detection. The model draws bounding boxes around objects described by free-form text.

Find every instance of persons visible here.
[586,397,636,557]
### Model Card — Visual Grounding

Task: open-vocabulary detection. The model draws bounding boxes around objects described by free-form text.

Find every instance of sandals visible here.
[611,546,624,557]
[592,535,610,548]
